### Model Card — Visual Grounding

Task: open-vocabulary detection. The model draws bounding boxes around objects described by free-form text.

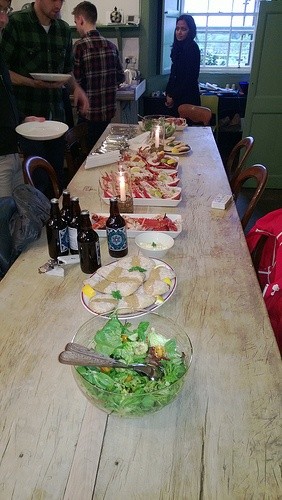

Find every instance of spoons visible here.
[65,343,155,380]
[58,350,164,382]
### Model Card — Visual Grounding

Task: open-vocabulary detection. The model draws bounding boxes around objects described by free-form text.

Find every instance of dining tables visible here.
[1,122,282,500]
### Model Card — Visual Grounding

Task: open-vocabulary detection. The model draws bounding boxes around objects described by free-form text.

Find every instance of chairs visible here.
[22,154,61,214]
[250,209,282,339]
[226,135,255,187]
[199,94,220,146]
[230,163,269,231]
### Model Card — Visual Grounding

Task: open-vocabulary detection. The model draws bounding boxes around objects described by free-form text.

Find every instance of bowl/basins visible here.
[134,232,174,258]
[66,307,193,420]
[141,114,177,138]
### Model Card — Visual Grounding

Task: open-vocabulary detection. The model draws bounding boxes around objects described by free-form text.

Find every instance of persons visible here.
[2,0,91,199]
[69,1,126,162]
[0,0,26,196]
[165,15,203,127]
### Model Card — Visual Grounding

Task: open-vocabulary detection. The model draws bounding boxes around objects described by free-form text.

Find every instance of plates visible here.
[80,256,178,321]
[14,120,68,141]
[102,142,193,207]
[30,72,73,81]
[87,212,183,238]
[175,123,188,131]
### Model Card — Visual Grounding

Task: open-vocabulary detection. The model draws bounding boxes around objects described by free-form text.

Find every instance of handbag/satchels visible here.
[8,184,51,256]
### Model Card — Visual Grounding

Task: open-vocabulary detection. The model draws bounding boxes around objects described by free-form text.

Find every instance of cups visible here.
[150,118,165,152]
[108,164,134,213]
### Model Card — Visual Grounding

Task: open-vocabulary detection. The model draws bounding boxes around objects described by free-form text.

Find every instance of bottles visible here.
[68,195,85,254]
[58,188,76,248]
[106,197,128,258]
[46,199,69,260]
[77,209,101,274]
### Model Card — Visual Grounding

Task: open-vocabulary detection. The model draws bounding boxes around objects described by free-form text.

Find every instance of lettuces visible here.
[82,314,185,418]
